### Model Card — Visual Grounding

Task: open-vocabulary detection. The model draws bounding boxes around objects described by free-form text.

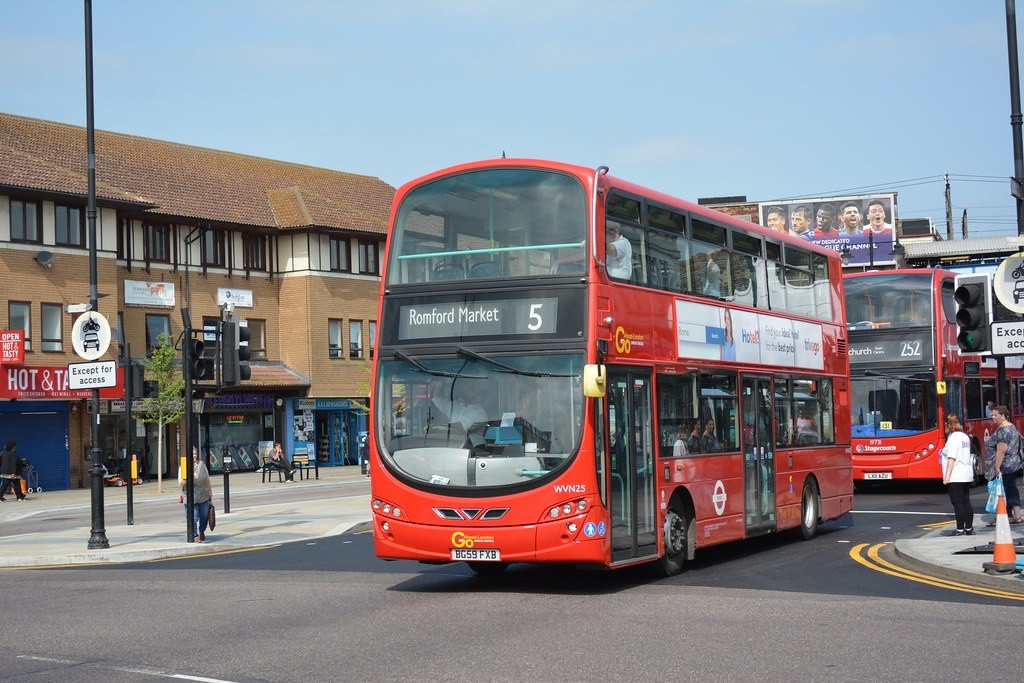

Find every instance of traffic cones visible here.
[982,494,1018,571]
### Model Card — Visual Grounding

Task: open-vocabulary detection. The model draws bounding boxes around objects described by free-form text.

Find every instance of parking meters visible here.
[222,444,232,513]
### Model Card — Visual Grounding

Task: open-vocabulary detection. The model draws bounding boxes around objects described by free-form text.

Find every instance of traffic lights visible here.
[190,338,206,379]
[953,274,992,356]
[222,322,252,388]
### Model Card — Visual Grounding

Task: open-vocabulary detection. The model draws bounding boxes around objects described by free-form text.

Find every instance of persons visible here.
[362,432,369,474]
[985,406,1024,527]
[767,200,893,262]
[551,221,632,281]
[434,376,488,433]
[178,447,213,543]
[0,440,26,502]
[705,249,720,295]
[268,443,297,482]
[941,414,975,536]
[745,403,829,458]
[673,418,719,456]
[986,401,994,418]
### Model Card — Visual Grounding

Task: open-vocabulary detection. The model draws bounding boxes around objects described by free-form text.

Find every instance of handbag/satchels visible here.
[985,472,1007,513]
[208,504,216,531]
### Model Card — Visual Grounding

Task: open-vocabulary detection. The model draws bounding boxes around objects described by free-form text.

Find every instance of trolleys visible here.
[21,457,43,494]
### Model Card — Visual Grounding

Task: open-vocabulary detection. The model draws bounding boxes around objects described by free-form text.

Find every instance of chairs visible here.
[262,454,319,483]
[432,253,670,289]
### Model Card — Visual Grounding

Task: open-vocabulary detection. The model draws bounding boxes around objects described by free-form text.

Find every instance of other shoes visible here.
[194,536,202,542]
[964,526,975,535]
[289,469,296,474]
[0,497,7,503]
[947,529,967,536]
[199,531,205,541]
[17,494,26,501]
[285,479,294,483]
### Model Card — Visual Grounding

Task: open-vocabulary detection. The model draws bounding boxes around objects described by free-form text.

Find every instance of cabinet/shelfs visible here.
[318,435,329,462]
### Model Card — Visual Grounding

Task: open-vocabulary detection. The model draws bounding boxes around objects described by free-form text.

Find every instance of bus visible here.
[843,266,1023,488]
[370,155,854,579]
